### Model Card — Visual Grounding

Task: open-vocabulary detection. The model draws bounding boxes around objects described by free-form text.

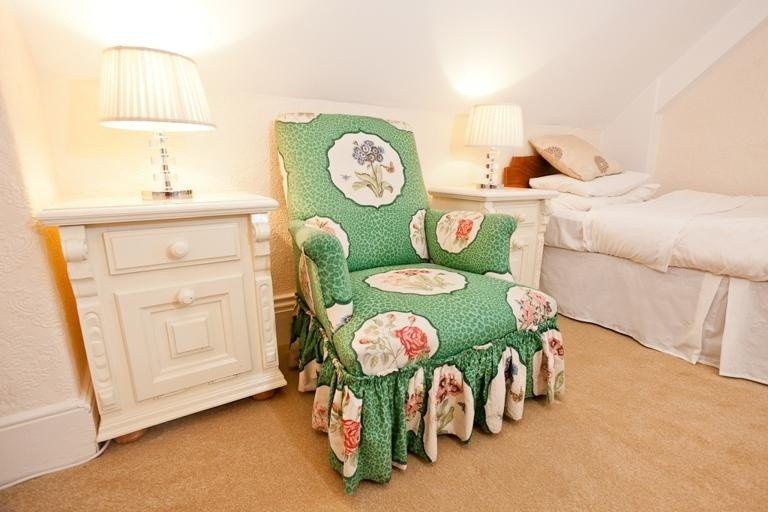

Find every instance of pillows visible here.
[528,171,660,210]
[529,133,625,181]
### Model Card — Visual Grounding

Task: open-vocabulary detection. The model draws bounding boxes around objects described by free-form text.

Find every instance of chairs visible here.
[275,113,567,493]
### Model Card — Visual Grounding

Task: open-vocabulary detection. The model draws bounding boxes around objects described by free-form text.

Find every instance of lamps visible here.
[98,45,216,201]
[464,104,524,189]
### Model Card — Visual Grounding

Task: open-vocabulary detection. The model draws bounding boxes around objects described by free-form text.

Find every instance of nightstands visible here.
[427,186,559,289]
[35,193,287,444]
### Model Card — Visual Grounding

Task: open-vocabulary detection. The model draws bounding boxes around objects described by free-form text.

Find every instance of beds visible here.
[504,156,768,384]
